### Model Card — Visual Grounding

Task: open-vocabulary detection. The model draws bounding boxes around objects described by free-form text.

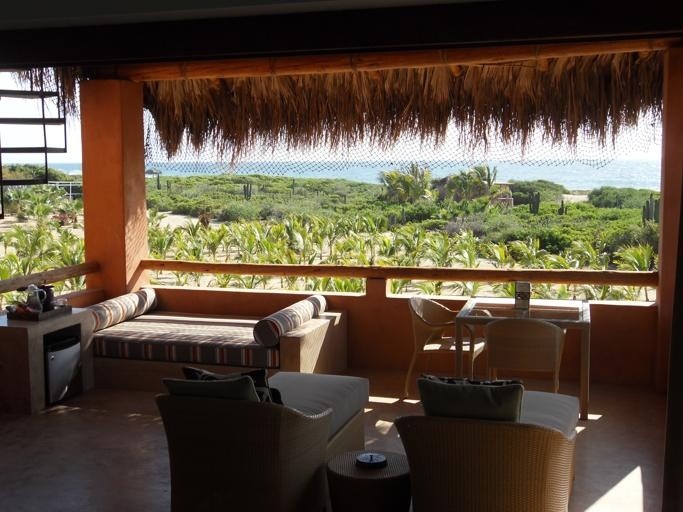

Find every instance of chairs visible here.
[402,298,487,397]
[486,319,566,396]
[151,367,369,511]
[393,372,579,512]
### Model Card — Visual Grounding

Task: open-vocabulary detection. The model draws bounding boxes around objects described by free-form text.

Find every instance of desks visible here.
[455,298,590,422]
[1,306,95,415]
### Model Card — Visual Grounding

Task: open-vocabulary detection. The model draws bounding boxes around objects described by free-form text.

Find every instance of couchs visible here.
[83,289,349,393]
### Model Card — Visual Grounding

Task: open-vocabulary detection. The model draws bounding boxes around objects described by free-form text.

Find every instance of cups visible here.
[52,297,68,310]
[515,282,530,310]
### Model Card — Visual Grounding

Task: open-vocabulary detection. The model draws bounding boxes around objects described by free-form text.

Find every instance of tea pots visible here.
[20,283,47,318]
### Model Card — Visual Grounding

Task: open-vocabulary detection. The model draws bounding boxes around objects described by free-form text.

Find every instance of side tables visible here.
[326,450,411,511]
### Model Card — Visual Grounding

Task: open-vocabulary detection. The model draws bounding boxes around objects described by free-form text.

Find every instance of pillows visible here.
[415,374,523,421]
[162,367,282,404]
